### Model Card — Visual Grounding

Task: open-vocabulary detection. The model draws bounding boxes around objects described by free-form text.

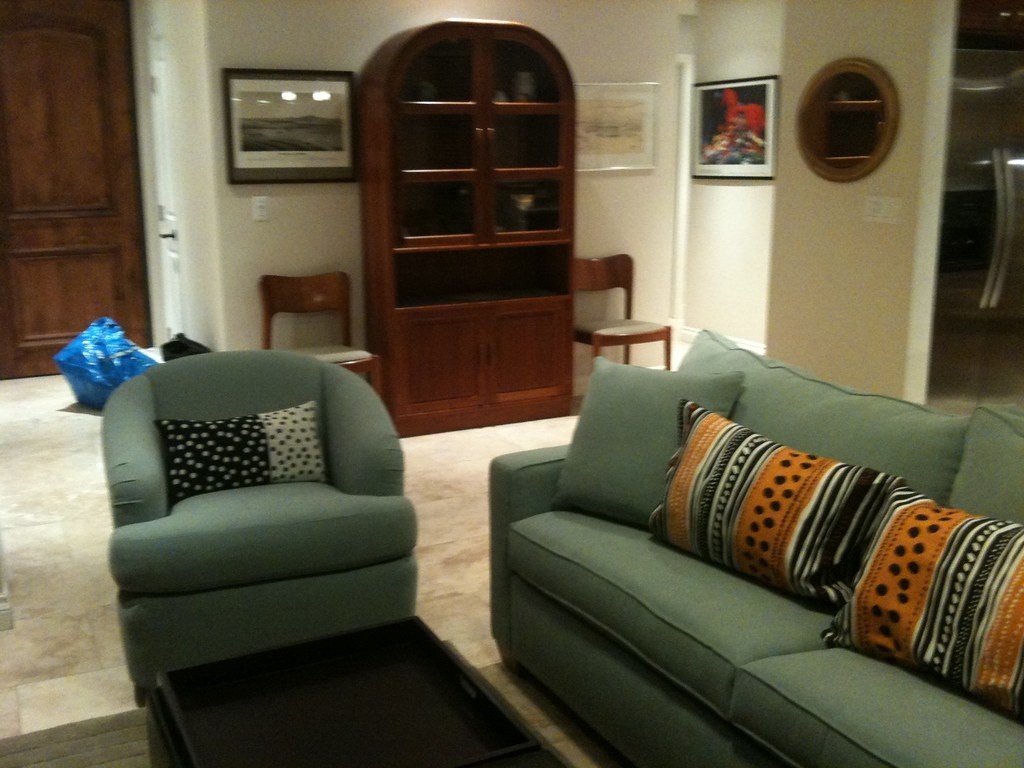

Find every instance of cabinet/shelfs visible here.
[356,22,576,438]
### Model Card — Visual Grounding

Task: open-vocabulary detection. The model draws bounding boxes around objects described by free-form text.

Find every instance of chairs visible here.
[257,271,382,393]
[576,254,671,371]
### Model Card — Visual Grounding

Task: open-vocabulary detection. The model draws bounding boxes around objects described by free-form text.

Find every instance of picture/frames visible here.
[690,75,776,181]
[573,82,658,174]
[221,67,358,185]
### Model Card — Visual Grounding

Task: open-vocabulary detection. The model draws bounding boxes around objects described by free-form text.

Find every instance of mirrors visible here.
[793,58,899,184]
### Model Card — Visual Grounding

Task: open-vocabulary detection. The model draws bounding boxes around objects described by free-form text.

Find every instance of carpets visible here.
[0,661,628,768]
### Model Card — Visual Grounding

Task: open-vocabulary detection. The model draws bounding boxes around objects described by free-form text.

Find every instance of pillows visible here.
[649,398,907,609]
[943,404,1024,523]
[820,481,1024,725]
[548,357,745,533]
[676,328,969,507]
[152,400,327,507]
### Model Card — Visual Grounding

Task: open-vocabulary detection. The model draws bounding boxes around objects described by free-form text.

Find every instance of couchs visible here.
[99,350,419,708]
[489,327,1024,768]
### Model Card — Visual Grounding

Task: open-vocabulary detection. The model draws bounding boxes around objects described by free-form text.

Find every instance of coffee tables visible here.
[146,615,572,768]
